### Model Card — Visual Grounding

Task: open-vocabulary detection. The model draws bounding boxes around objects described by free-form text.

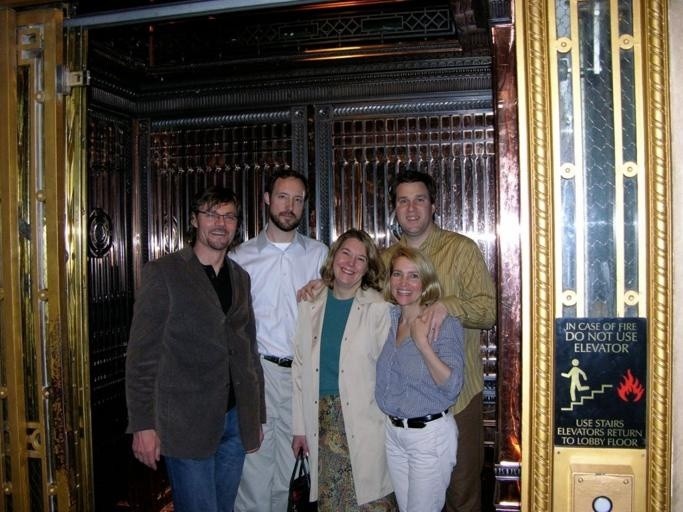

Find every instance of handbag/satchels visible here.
[286,448,318,512]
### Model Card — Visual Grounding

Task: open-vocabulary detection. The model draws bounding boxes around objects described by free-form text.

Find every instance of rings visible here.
[431,328,435,331]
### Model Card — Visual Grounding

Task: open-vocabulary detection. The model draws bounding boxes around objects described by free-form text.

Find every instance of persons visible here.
[224,169,331,512]
[375,247,466,511]
[297,170,496,512]
[124,185,266,512]
[290,227,398,512]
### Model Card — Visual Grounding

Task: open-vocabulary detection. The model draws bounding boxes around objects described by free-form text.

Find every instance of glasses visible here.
[196,208,237,223]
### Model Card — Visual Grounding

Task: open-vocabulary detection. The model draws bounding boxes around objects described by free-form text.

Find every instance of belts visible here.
[262,355,292,368]
[390,409,449,429]
